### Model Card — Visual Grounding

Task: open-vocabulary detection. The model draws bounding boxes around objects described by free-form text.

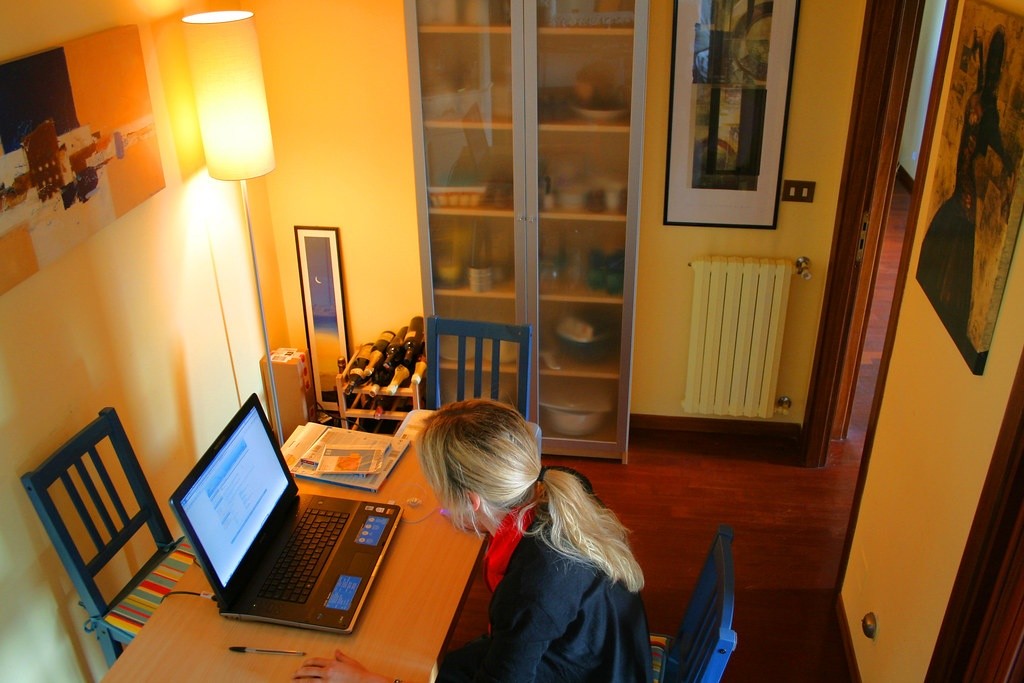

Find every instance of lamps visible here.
[180,8,283,448]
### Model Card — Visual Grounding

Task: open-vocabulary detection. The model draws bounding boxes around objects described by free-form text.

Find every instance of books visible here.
[283,422,412,493]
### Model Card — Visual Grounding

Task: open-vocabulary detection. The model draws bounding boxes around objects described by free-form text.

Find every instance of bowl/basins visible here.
[545,405,604,435]
[556,321,614,362]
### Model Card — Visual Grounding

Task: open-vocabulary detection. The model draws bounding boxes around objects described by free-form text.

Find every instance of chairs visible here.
[20,407,198,669]
[650,524,738,683]
[426,316,533,423]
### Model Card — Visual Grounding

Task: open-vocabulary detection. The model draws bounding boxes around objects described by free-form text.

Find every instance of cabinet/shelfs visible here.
[403,0,651,468]
[335,350,420,437]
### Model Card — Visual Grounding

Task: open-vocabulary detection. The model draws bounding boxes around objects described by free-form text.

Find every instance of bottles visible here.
[338,316,428,419]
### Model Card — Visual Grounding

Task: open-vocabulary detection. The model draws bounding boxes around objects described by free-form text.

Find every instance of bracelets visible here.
[394,679,401,683]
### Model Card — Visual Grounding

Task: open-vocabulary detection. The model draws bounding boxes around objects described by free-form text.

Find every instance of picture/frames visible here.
[294,226,351,413]
[662,0,801,230]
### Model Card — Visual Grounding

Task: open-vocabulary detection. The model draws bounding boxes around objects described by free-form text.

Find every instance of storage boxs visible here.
[426,183,486,207]
[259,349,317,445]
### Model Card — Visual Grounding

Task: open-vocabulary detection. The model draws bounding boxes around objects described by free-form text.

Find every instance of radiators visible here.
[682,255,811,419]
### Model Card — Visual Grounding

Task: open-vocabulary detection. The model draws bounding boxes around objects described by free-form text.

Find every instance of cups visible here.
[607,192,621,213]
[466,265,493,292]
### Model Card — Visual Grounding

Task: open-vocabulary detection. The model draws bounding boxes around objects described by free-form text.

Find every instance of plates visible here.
[482,339,516,363]
[570,103,624,121]
[438,333,477,360]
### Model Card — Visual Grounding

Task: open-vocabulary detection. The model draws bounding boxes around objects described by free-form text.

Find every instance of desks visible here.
[97,422,488,683]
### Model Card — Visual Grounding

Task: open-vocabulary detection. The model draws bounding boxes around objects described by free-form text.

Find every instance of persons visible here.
[290,398,654,683]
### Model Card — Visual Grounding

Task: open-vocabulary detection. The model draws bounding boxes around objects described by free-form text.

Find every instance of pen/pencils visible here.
[229,646,306,657]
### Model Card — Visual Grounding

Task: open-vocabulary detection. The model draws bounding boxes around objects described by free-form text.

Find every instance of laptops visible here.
[167,392,403,634]
